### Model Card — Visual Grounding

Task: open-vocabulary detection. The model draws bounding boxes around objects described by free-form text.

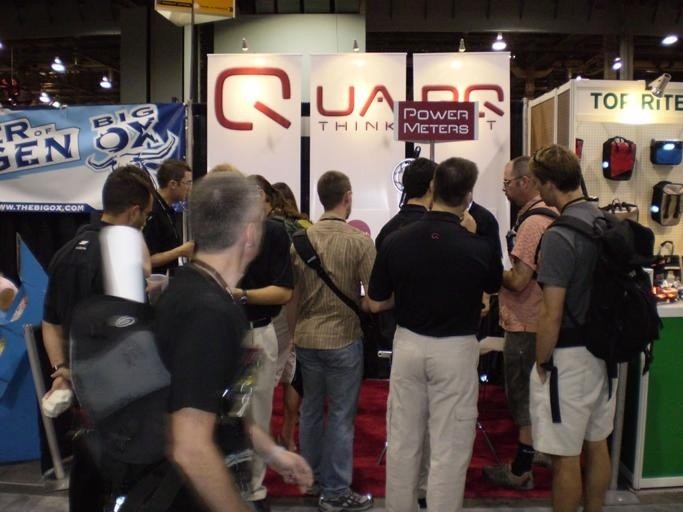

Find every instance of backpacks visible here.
[66,293,171,512]
[533,207,663,424]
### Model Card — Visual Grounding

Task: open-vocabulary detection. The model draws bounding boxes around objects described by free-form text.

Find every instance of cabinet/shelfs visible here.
[615,297,683,490]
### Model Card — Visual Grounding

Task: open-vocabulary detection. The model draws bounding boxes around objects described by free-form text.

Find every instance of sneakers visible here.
[482,462,537,492]
[318,489,374,511]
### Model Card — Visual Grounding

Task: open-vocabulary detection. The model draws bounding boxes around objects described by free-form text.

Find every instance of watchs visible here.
[48,363,73,376]
[239,289,248,306]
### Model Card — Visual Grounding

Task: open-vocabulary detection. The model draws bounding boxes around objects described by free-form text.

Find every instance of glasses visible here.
[174,179,194,187]
[502,175,524,185]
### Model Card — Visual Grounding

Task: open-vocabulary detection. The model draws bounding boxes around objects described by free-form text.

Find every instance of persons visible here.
[272,181,297,211]
[374,157,440,509]
[527,142,625,510]
[362,157,505,512]
[142,156,196,278]
[480,155,559,491]
[247,173,311,227]
[208,164,295,512]
[147,165,314,512]
[291,169,379,512]
[41,162,166,512]
[462,194,500,316]
